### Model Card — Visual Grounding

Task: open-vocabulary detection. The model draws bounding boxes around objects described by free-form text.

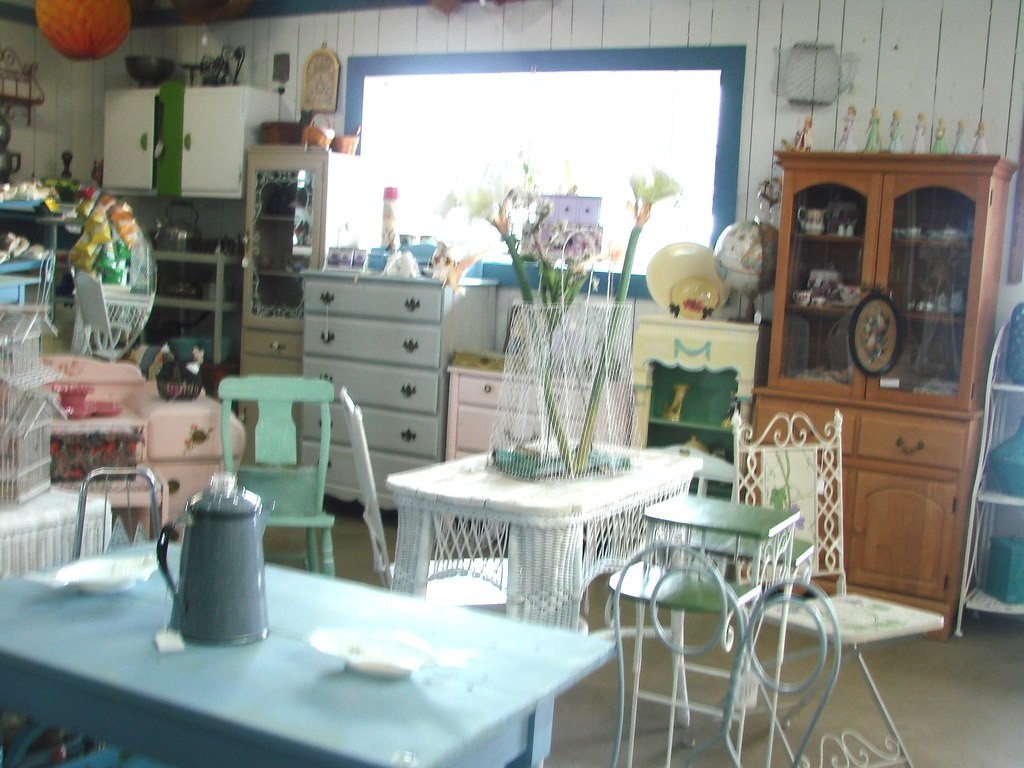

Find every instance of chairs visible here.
[338,386,509,611]
[218,375,337,575]
[731,408,945,768]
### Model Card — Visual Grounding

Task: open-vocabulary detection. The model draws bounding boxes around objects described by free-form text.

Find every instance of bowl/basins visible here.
[125,56,174,89]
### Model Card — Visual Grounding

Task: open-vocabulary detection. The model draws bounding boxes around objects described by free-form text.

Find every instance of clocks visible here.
[301,42,340,112]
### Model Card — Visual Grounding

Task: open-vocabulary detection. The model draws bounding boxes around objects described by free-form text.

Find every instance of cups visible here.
[837,287,860,302]
[894,225,967,242]
[792,290,811,305]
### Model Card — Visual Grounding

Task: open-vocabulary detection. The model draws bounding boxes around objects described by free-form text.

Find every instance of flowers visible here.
[440,151,684,479]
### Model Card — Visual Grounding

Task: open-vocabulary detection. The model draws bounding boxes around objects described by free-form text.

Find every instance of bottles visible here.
[380,186,399,250]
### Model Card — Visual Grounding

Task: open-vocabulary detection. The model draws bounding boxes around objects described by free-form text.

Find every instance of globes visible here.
[713,215,779,325]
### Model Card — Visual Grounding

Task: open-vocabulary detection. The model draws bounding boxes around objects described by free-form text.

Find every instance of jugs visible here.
[155,471,276,647]
[797,208,827,235]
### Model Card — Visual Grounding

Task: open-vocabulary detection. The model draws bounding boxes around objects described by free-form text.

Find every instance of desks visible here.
[610,494,814,768]
[385,442,702,768]
[0,540,618,768]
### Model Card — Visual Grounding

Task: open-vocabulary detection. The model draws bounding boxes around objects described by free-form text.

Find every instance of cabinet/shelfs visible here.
[631,314,771,582]
[955,323,1024,638]
[741,150,1017,648]
[446,367,631,462]
[238,146,385,466]
[0,199,243,365]
[301,271,500,510]
[101,86,280,199]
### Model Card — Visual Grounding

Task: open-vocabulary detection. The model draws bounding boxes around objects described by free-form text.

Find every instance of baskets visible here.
[155,375,202,400]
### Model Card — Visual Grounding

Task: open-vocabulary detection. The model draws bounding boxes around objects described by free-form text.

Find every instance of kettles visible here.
[154,201,202,252]
